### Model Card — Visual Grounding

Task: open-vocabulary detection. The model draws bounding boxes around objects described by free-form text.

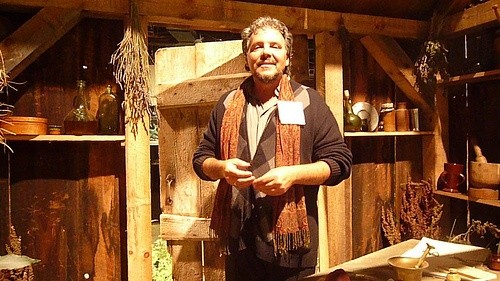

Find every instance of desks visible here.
[304,239,500,281]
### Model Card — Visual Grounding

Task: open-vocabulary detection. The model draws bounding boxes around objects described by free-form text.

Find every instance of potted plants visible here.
[473,220,500,271]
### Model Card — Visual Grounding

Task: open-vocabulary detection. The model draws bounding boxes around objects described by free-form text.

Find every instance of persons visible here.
[192,17,352,281]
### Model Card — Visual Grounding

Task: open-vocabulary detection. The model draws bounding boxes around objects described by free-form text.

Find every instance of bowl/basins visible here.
[470,161,500,190]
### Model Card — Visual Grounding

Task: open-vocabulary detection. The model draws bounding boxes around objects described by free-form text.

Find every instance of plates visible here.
[352,101,379,131]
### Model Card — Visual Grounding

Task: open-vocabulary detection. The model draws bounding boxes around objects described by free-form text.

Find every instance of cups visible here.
[440,163,466,193]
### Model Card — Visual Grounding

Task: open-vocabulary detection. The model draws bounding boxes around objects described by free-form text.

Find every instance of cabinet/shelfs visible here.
[436,70,500,208]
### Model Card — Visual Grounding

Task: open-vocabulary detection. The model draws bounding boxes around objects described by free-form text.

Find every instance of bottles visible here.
[395,102,409,131]
[63,80,99,134]
[447,268,461,281]
[96,84,120,134]
[380,103,396,132]
[343,90,362,132]
[388,256,429,281]
